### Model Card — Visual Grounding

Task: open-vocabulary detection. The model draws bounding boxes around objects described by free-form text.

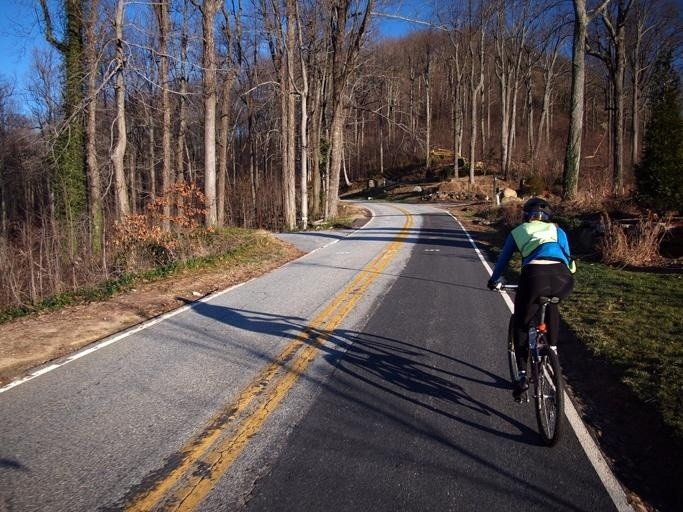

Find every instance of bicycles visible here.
[488,279,566,450]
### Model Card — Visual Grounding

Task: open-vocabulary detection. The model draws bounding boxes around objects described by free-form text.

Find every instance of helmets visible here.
[521,198,554,222]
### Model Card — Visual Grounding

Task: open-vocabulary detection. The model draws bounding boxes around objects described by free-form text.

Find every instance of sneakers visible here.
[512,376,530,398]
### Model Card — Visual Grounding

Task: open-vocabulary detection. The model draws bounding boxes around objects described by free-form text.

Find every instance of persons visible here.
[486,197,579,401]
